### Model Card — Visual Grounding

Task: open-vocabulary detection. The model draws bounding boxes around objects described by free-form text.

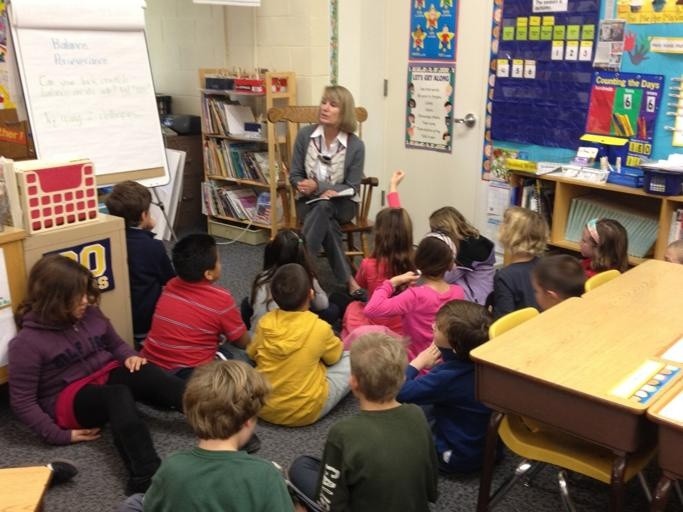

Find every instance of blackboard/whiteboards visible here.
[7,4,171,192]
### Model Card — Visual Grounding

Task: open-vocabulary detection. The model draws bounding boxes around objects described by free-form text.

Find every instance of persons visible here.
[484,206,551,320]
[244,263,352,427]
[363,230,465,378]
[7,253,262,498]
[579,218,629,278]
[530,254,585,311]
[284,332,441,512]
[118,358,308,512]
[340,169,418,342]
[288,86,370,303]
[430,207,497,307]
[138,233,256,379]
[664,240,683,264]
[395,299,505,478]
[104,180,177,351]
[240,226,340,332]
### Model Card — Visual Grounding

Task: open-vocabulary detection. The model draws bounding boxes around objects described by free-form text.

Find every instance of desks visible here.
[0,466,55,511]
[0,224,28,384]
[469,259,683,512]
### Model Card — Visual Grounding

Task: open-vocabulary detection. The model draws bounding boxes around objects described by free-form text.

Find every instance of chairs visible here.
[267,105,379,271]
[584,270,621,293]
[488,307,683,512]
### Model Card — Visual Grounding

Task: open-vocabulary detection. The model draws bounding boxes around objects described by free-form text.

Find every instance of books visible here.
[566,193,596,243]
[201,179,292,228]
[626,207,660,258]
[15,157,97,230]
[669,208,683,243]
[596,200,626,231]
[509,176,555,227]
[506,158,563,175]
[202,93,266,140]
[203,137,292,186]
[0,157,23,229]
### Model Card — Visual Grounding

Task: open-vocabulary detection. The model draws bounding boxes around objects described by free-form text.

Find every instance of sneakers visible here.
[44,458,80,487]
[239,435,262,455]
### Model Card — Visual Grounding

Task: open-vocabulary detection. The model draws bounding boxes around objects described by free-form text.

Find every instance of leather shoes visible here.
[344,282,368,303]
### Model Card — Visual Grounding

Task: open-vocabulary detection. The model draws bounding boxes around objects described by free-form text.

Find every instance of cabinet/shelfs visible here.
[22,213,135,351]
[509,169,682,267]
[164,135,207,227]
[198,66,297,240]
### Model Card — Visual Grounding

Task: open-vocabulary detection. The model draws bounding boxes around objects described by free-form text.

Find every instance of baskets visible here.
[603,163,647,189]
[643,167,683,196]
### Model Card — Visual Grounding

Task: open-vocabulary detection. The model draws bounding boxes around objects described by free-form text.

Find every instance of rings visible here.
[301,189,304,194]
